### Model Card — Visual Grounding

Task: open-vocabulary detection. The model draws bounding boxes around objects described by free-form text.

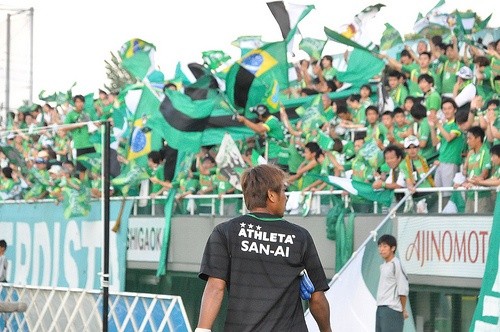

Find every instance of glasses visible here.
[406,146,419,149]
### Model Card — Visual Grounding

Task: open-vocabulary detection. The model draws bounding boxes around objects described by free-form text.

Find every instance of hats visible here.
[455,66,473,79]
[249,105,269,116]
[48,164,62,176]
[403,135,419,149]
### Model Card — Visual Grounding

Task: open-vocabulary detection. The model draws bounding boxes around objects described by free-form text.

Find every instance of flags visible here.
[109,1,495,169]
[305,171,394,209]
[303,218,415,332]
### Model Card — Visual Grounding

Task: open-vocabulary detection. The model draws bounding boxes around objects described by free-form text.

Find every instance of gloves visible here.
[300,269,315,300]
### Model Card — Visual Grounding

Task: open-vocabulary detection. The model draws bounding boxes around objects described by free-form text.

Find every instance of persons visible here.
[376,234,409,332]
[195,164,331,332]
[1,27,500,216]
[0,240,8,282]
